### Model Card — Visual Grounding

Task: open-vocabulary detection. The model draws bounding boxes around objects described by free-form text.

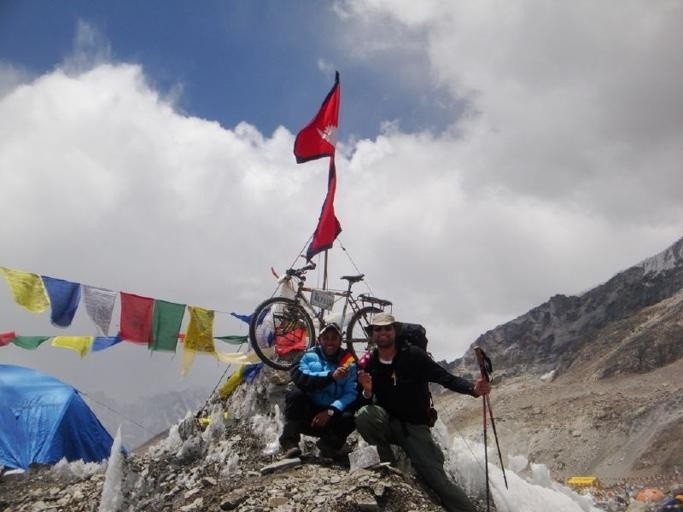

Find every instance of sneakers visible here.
[283,443,301,458]
[319,448,343,458]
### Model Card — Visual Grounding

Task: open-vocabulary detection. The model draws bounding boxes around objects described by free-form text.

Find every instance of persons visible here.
[354,312,491,511]
[276,320,362,468]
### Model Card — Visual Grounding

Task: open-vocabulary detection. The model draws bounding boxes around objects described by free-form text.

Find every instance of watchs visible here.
[326,408,334,418]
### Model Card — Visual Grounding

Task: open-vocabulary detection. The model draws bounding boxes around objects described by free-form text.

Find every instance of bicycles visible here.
[250,254,395,370]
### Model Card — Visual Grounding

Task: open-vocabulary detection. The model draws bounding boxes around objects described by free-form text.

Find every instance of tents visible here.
[0,363,128,474]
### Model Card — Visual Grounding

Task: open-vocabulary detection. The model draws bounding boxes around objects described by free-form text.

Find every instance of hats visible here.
[364,314,403,335]
[318,323,343,338]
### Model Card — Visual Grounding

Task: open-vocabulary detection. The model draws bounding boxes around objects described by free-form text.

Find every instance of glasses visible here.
[374,326,392,332]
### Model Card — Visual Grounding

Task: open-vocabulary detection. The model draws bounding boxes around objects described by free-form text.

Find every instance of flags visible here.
[292,80,340,266]
